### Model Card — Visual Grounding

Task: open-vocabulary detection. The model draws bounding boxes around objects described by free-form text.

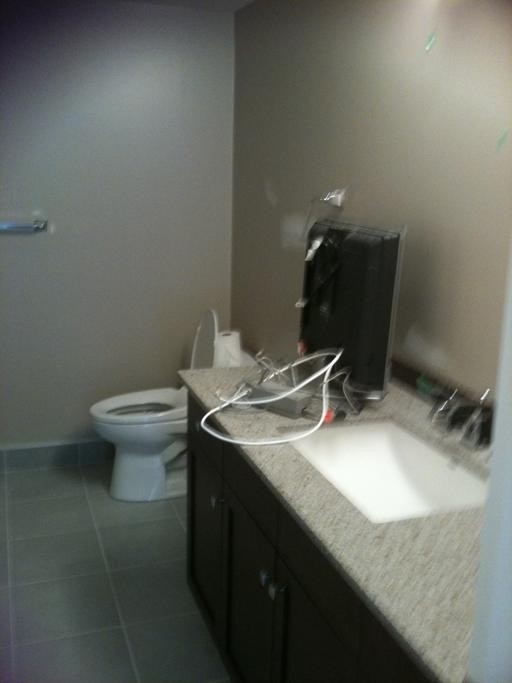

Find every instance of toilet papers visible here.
[218,330,241,368]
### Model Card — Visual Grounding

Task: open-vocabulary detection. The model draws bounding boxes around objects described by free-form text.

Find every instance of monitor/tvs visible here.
[296,215,410,398]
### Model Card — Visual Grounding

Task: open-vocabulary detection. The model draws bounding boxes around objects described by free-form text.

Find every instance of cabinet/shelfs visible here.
[184,391,440,683]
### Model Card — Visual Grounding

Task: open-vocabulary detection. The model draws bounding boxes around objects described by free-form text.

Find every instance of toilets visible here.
[90,309,260,502]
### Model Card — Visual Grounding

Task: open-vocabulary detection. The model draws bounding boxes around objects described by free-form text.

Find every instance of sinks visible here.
[276,412,492,526]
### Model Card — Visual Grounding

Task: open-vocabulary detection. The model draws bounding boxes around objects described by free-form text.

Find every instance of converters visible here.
[246,377,314,418]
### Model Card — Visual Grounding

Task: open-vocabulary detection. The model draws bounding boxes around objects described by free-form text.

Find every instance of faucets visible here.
[432,394,478,428]
